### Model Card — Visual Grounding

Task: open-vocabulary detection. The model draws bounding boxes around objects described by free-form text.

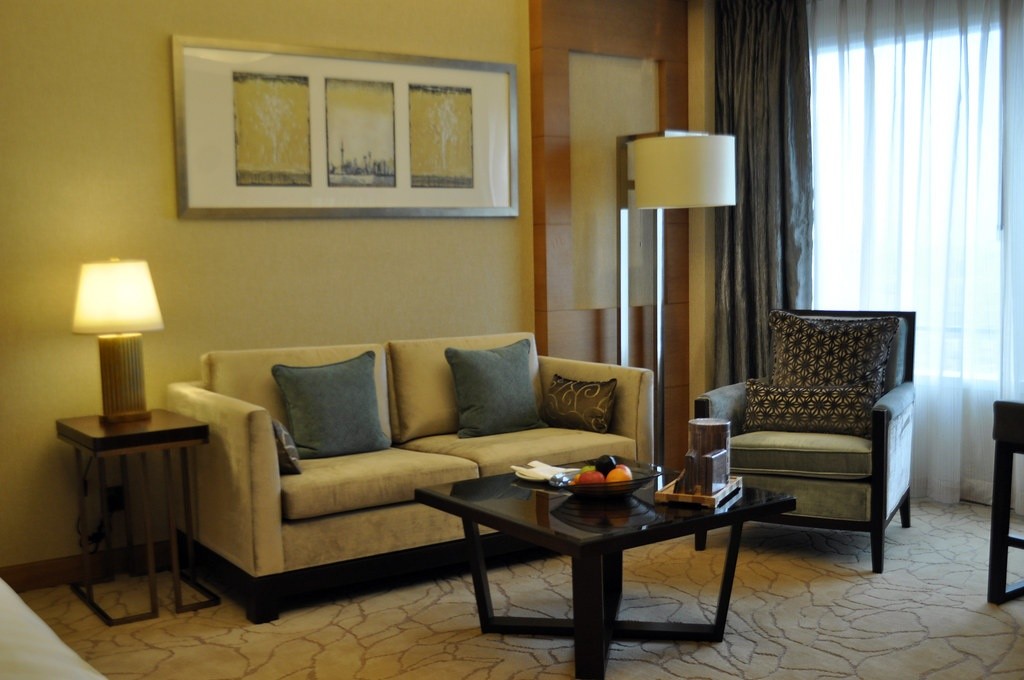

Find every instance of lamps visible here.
[71,260,164,425]
[616,130,737,465]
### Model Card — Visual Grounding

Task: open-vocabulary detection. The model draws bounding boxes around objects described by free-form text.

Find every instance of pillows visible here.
[444,339,548,439]
[768,310,899,402]
[541,373,618,433]
[270,351,390,459]
[273,419,301,475]
[742,379,876,439]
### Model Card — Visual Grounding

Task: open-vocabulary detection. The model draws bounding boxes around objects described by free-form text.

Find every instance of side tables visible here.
[57,409,223,627]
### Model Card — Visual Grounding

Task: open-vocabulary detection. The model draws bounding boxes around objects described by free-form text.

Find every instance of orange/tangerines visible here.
[606,468,631,482]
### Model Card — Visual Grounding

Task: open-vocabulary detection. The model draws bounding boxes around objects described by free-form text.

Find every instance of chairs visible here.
[693,309,917,574]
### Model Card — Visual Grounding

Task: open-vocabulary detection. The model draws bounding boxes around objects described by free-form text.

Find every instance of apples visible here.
[579,470,604,482]
[616,465,629,470]
[580,466,595,474]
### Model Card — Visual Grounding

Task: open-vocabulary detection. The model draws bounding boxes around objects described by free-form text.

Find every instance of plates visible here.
[515,465,565,481]
[549,457,665,498]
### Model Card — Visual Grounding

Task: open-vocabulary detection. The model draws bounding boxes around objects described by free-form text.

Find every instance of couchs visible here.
[168,332,654,625]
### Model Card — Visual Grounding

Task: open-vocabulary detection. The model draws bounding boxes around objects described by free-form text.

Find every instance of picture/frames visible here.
[171,33,520,219]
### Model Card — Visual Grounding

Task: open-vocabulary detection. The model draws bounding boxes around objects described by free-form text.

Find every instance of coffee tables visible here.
[413,457,797,680]
